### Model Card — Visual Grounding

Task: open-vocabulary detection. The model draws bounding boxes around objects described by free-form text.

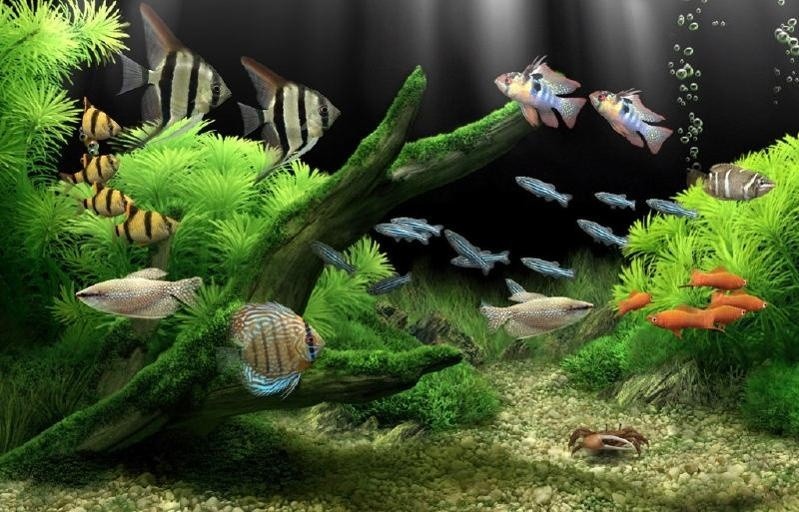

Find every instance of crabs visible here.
[567,423,650,458]
